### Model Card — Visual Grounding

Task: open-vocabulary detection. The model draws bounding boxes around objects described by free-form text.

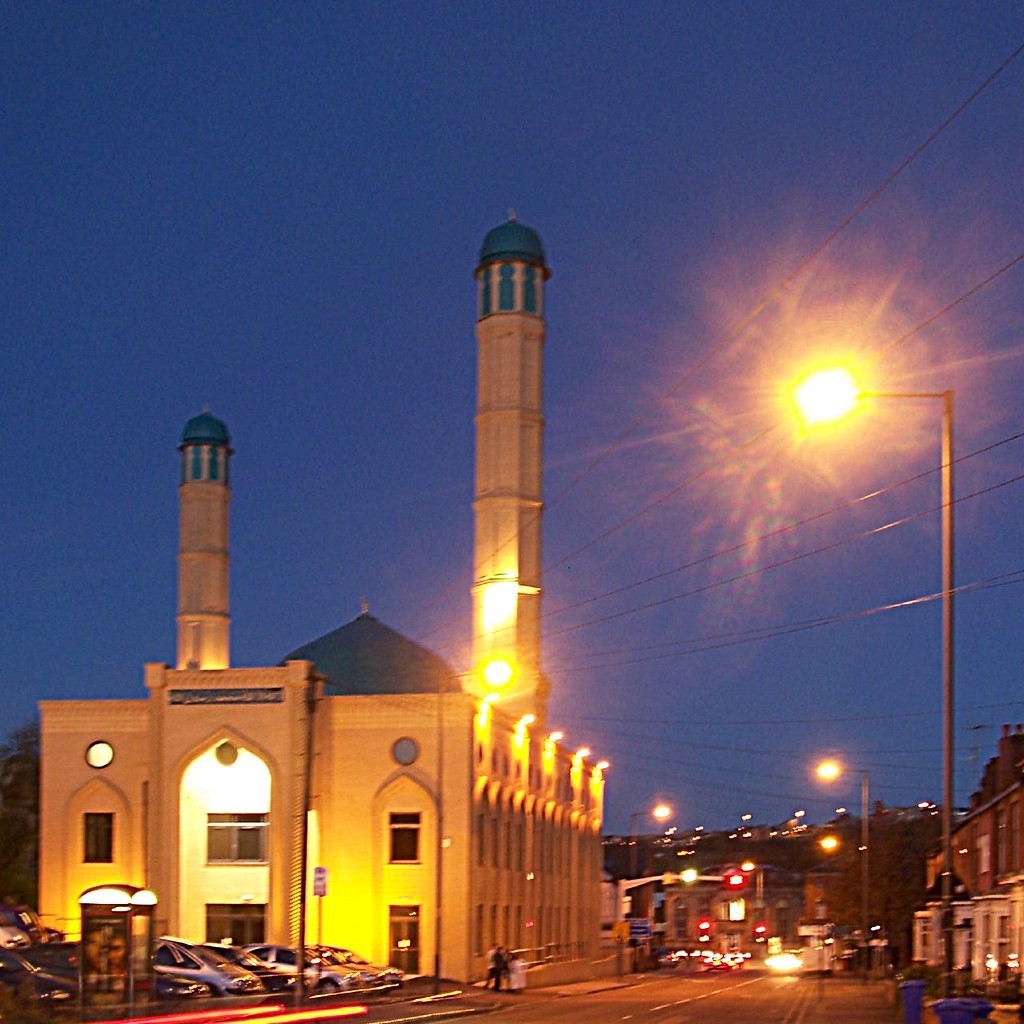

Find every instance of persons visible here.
[483,942,527,994]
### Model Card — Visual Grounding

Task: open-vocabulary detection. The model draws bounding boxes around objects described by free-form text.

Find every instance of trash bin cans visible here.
[897,980,927,1024]
[933,998,995,1024]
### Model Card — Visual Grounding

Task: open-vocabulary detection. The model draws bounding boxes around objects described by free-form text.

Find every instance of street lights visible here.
[793,369,953,1002]
[431,658,513,998]
[630,805,671,918]
[817,761,868,984]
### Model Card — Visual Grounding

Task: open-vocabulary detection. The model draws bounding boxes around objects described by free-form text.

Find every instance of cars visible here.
[0,906,404,1013]
